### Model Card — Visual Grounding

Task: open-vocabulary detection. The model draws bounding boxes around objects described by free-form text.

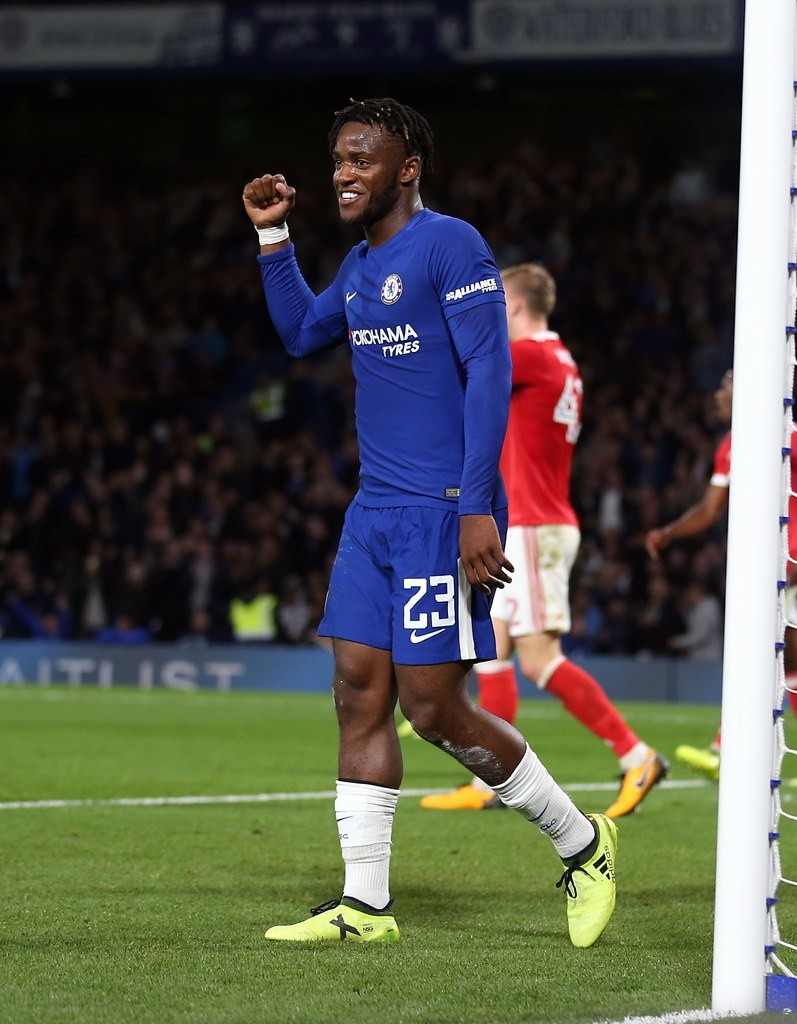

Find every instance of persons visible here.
[243,96,620,947]
[0,64,797,816]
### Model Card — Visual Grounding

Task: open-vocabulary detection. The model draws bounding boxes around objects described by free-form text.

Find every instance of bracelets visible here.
[253,221,289,245]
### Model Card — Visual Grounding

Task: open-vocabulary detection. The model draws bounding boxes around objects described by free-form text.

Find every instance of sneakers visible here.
[420,783,507,809]
[265,892,399,944]
[604,747,670,818]
[556,813,618,948]
[676,745,720,784]
